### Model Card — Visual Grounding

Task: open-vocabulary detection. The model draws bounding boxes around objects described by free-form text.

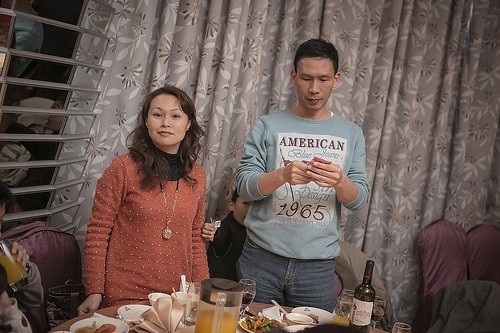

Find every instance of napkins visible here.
[131,297,188,333]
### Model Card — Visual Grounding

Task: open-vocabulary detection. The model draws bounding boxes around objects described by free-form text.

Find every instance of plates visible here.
[282,325,316,333]
[69,317,129,333]
[291,307,333,325]
[117,305,152,323]
[238,317,288,333]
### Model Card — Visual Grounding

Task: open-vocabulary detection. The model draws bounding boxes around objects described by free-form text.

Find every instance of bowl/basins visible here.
[285,313,313,325]
[148,293,171,305]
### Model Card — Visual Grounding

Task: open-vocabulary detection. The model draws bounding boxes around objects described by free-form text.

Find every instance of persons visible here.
[238,38,370,314]
[76,85,210,317]
[0,182,44,333]
[201,186,251,283]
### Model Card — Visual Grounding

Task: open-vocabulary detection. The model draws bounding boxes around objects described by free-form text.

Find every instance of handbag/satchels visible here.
[0,141,31,187]
[44,280,87,330]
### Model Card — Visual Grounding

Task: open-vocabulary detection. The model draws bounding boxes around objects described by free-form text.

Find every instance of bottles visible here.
[349,260,375,333]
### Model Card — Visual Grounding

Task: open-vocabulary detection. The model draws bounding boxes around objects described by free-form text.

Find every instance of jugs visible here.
[195,278,244,333]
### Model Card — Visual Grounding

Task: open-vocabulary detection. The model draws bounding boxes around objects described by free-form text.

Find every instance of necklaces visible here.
[158,178,179,239]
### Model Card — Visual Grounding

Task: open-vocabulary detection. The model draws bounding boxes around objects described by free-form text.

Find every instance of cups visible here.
[392,321,411,333]
[332,289,354,327]
[0,239,29,293]
[171,282,201,326]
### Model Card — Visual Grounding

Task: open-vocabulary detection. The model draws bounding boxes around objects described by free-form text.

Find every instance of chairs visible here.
[334,218,500,333]
[15,227,83,299]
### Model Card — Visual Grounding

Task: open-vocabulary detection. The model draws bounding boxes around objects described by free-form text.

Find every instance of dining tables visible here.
[50,301,387,333]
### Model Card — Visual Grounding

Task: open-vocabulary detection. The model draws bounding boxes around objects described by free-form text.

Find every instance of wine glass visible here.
[238,278,256,319]
[371,296,386,329]
